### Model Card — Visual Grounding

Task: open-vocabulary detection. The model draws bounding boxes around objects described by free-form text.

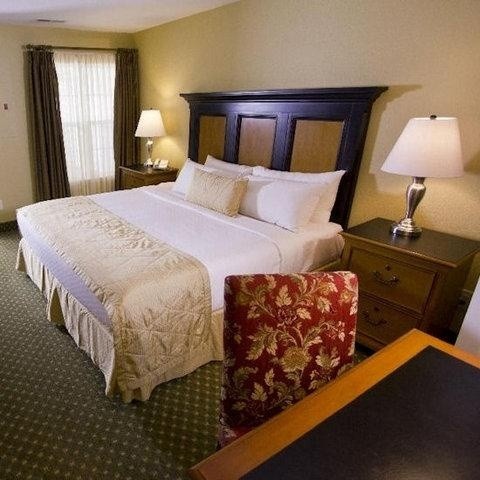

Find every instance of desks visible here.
[189,326,480,480]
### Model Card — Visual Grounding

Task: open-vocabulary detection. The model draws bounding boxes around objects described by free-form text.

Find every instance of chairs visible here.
[217,270,359,449]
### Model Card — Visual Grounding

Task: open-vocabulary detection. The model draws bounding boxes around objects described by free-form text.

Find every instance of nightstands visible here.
[338,217,480,352]
[119,164,179,190]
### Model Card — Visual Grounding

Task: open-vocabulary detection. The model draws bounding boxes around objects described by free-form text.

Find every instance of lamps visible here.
[134,108,166,166]
[381,114,466,237]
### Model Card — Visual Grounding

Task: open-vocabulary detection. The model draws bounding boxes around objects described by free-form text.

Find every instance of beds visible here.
[13,84,388,406]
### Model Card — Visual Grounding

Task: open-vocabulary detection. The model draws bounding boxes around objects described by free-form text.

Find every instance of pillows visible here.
[204,154,253,178]
[184,168,249,218]
[252,164,348,229]
[238,171,326,233]
[172,158,243,200]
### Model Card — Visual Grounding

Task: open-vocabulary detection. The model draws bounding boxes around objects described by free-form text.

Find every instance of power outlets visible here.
[0,200,3,211]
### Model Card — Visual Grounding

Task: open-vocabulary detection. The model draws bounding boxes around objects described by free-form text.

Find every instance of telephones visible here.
[153,158,169,170]
